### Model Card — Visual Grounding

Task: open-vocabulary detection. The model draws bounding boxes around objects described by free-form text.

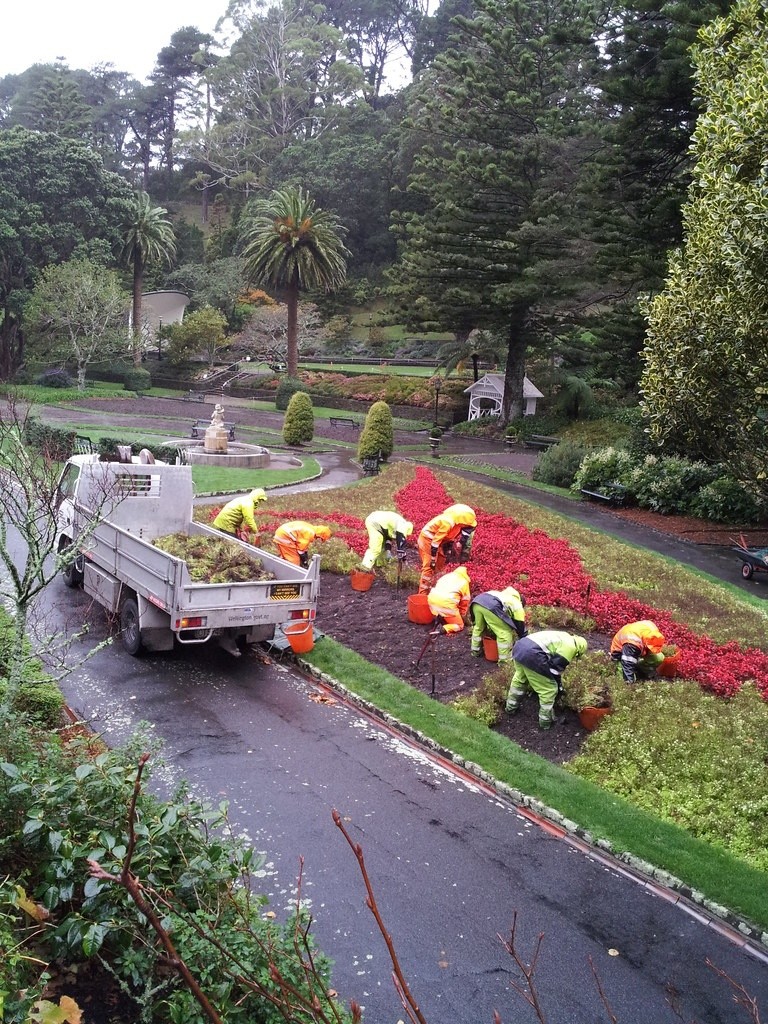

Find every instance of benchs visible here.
[525,434,559,449]
[329,417,360,431]
[192,419,236,442]
[581,482,628,510]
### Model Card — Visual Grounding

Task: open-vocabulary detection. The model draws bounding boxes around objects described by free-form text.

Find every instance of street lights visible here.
[157,315,163,361]
[432,377,441,428]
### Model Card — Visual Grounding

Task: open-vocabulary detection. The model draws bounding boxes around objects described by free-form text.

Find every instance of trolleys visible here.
[732,543,768,581]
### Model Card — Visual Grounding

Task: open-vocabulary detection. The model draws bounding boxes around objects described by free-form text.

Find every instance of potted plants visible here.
[505,426,517,444]
[429,428,442,447]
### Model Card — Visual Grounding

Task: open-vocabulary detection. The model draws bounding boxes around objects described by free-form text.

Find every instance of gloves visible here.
[301,557,309,569]
[445,555,456,564]
[459,556,468,563]
[559,683,566,694]
[387,553,392,561]
[430,560,436,570]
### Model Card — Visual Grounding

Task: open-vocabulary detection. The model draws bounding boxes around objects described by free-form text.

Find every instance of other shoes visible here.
[471,650,482,657]
[431,624,445,639]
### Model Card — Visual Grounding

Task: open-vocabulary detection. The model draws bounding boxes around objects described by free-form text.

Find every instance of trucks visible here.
[49,445,322,659]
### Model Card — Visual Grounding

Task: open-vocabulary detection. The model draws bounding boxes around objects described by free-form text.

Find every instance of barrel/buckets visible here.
[655,645,684,677]
[351,568,374,591]
[407,594,434,625]
[579,707,612,732]
[283,619,315,654]
[482,633,498,663]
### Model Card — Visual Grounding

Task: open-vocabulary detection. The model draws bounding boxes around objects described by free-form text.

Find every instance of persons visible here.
[418,504,477,594]
[211,404,224,425]
[610,619,665,683]
[470,586,528,666]
[275,521,333,567]
[212,488,267,537]
[428,567,471,634]
[362,511,414,572]
[507,631,588,728]
